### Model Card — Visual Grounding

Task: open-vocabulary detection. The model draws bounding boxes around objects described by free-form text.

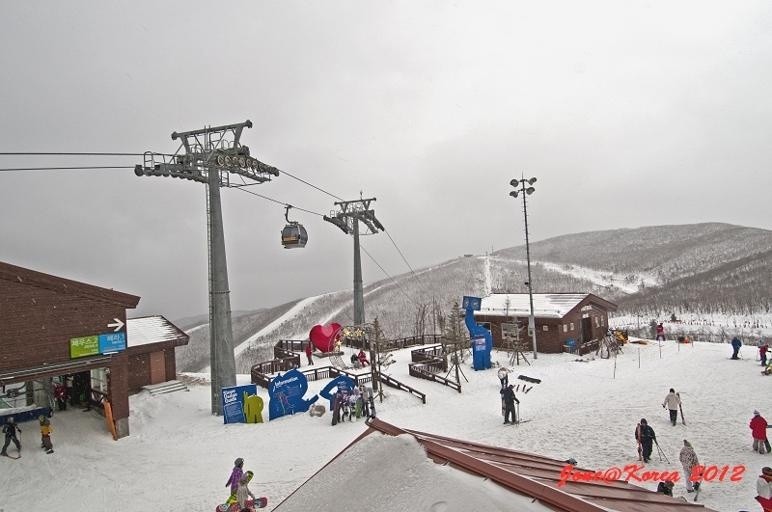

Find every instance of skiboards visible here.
[677,393,685,425]
[637,423,643,460]
[505,417,530,426]
[694,465,706,501]
[0,447,21,459]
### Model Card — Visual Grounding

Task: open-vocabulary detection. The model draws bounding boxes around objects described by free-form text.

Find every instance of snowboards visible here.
[216,498,267,512]
[224,472,253,506]
[764,438,771,452]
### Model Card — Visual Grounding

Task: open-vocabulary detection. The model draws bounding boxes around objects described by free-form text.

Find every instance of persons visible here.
[757,466,771,511]
[305,345,313,365]
[662,387,683,424]
[1,417,22,457]
[758,345,771,365]
[351,353,360,369]
[226,456,245,496]
[750,410,769,456]
[680,439,700,492]
[498,367,514,386]
[48,375,92,412]
[37,413,55,454]
[232,473,257,511]
[358,348,371,367]
[498,383,518,424]
[656,480,673,497]
[635,417,657,464]
[655,322,665,341]
[731,337,741,358]
